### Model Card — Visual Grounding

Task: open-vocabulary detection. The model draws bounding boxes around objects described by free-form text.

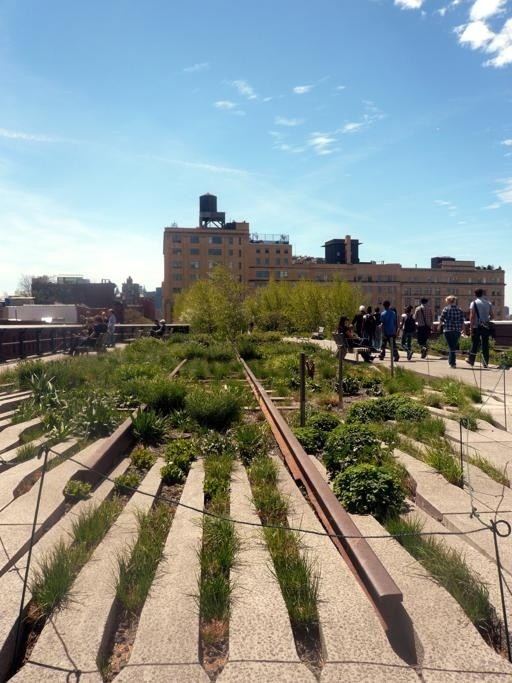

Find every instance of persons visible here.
[148,318,166,337]
[64,307,117,356]
[337,286,495,368]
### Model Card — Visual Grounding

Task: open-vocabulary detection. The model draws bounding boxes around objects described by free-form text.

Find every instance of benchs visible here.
[124,328,143,347]
[151,326,173,342]
[329,332,370,361]
[70,331,109,356]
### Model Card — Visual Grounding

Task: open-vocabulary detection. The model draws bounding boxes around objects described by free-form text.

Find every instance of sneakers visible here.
[378,345,476,368]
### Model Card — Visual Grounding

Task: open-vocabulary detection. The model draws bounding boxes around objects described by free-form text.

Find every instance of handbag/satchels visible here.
[347,338,367,346]
[478,318,494,335]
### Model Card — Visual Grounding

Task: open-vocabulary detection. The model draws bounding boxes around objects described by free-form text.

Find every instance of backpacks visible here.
[405,314,416,334]
